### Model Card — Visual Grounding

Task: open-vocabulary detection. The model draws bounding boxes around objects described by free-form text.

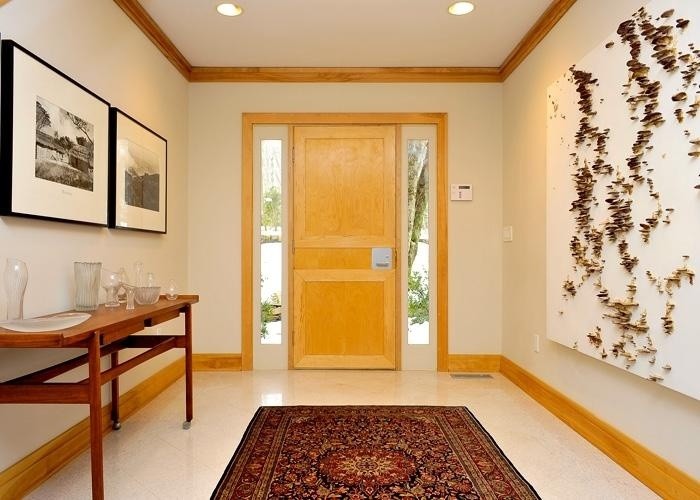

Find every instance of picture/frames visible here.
[1,39,167,239]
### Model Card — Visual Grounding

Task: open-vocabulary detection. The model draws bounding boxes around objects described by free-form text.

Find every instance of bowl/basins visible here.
[136,287,162,305]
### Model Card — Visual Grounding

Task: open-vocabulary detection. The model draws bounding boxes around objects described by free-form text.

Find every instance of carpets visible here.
[209,406,541,500]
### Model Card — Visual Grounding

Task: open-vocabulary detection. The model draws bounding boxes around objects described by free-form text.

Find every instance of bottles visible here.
[74,261,137,311]
[165,280,180,301]
[3,260,28,319]
[146,273,157,287]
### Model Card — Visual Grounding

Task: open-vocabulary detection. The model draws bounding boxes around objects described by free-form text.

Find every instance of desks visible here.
[0,296,199,500]
